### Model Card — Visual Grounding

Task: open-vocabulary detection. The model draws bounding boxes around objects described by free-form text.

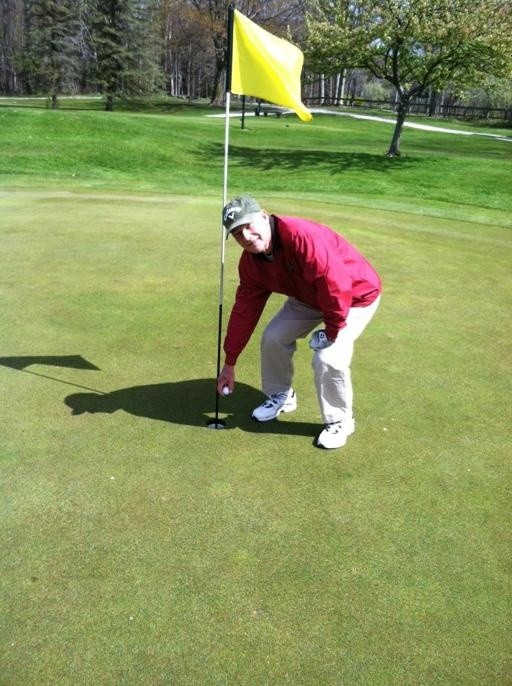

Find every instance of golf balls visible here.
[223,387,230,395]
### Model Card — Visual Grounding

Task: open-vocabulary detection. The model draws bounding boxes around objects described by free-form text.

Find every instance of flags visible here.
[231,7,315,125]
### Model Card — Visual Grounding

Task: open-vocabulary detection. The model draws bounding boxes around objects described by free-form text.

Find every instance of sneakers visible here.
[316,416,355,450]
[251,390,298,423]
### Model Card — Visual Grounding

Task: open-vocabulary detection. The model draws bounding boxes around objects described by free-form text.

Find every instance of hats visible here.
[221,195,262,241]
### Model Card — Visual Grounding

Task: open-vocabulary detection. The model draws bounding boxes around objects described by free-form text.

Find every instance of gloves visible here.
[308,330,334,352]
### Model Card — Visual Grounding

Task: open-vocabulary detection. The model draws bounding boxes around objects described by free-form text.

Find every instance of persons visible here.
[216,192,383,452]
